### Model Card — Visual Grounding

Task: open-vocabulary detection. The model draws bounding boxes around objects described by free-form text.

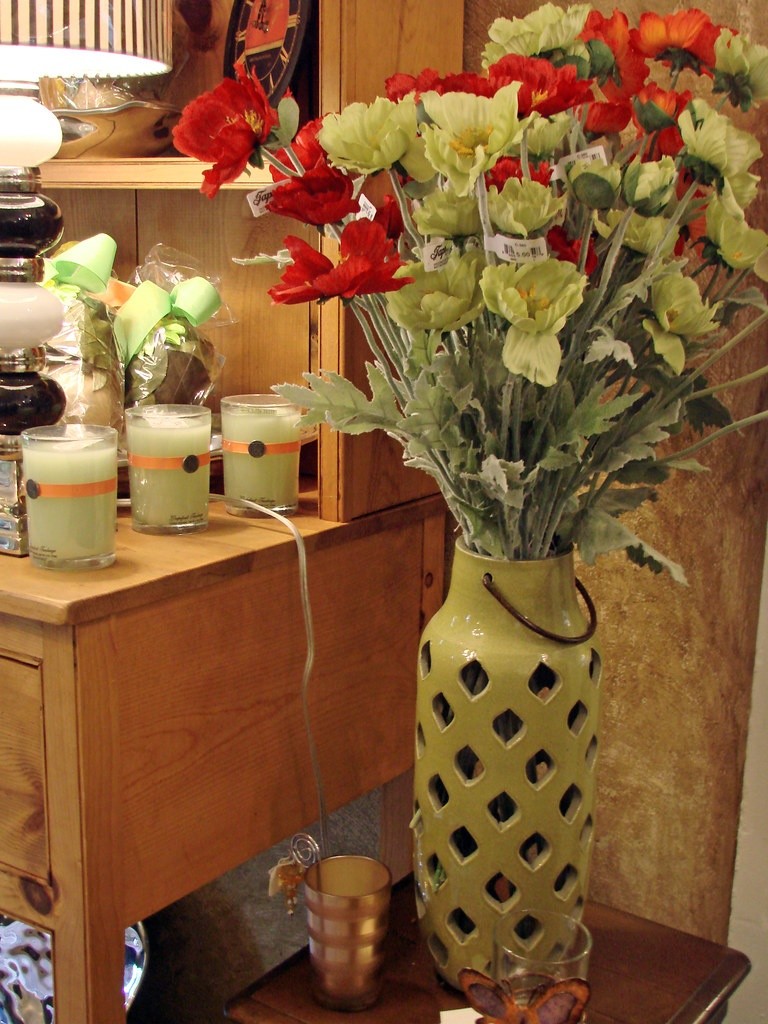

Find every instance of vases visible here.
[412,533,604,996]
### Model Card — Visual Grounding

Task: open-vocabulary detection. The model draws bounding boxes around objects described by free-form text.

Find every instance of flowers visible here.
[172,2,768,939]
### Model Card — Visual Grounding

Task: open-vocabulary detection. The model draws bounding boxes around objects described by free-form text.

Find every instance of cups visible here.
[23,424,118,571]
[491,908,593,1024]
[124,404,212,534]
[219,394,302,515]
[302,854,392,1009]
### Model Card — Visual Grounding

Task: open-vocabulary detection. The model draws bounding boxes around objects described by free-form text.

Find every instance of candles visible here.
[22,423,120,573]
[126,404,212,534]
[221,394,303,516]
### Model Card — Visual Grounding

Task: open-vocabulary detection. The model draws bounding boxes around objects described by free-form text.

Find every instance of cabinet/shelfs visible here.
[40,0,469,515]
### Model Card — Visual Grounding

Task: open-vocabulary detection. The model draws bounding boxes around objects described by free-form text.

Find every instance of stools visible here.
[227,871,753,1024]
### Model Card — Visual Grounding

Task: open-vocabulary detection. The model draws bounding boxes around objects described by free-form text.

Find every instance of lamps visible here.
[0,0,175,558]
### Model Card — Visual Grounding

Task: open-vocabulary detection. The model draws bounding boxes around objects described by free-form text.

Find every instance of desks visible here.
[0,495,448,1024]
